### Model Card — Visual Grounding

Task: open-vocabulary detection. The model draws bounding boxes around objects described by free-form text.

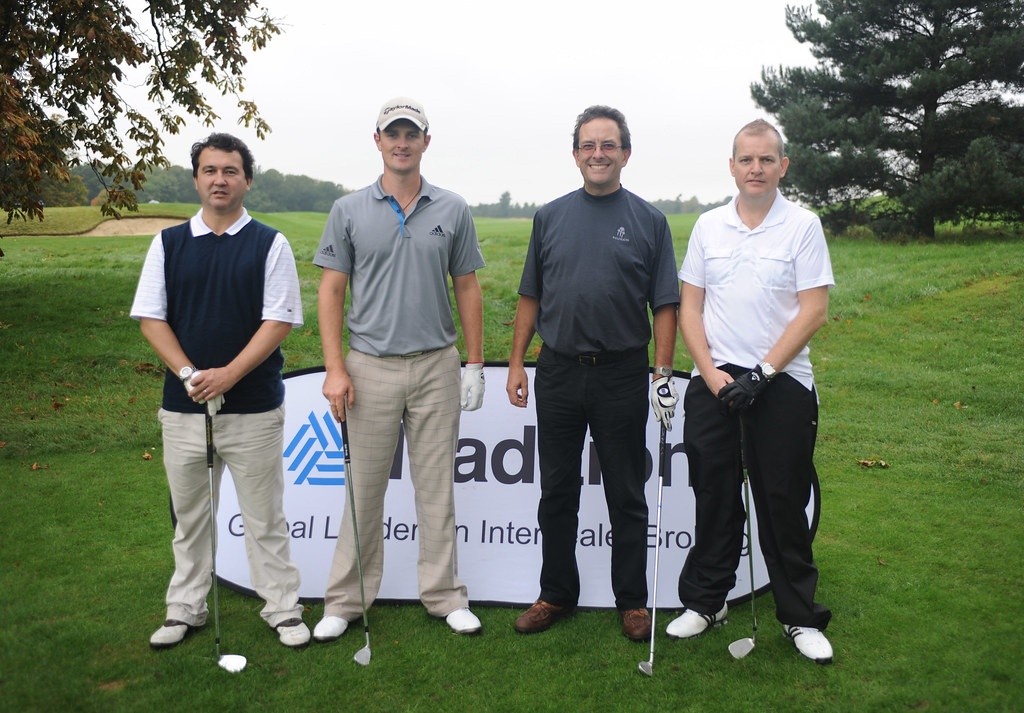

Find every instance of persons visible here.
[505,105,681,640]
[311,95,486,640]
[665,120,835,664]
[128,134,312,647]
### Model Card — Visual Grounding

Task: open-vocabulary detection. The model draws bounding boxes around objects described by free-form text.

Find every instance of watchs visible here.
[758,361,776,380]
[179,365,197,384]
[651,367,673,379]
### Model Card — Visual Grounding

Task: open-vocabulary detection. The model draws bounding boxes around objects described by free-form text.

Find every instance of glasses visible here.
[575,144,624,153]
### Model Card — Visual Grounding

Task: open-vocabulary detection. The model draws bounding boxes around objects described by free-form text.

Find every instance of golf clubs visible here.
[637,418,667,678]
[726,412,758,661]
[340,398,373,665]
[205,402,248,674]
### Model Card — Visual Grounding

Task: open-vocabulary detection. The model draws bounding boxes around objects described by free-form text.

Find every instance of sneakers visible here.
[782,622,833,663]
[620,606,651,641]
[150,619,201,649]
[513,598,577,632]
[312,615,349,641]
[273,617,310,648]
[444,608,481,634]
[665,600,728,638]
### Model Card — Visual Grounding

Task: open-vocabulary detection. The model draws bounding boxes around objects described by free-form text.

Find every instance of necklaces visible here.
[402,181,422,212]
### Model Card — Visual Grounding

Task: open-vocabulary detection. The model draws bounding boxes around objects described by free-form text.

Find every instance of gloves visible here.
[460,363,485,411]
[650,376,680,431]
[184,372,226,417]
[717,364,768,414]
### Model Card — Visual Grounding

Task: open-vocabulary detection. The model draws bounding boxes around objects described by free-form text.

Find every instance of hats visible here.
[375,97,430,133]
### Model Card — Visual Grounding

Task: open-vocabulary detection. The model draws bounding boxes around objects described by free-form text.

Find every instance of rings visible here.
[204,389,207,395]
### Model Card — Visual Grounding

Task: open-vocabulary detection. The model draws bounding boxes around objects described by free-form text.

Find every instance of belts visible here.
[578,352,637,366]
